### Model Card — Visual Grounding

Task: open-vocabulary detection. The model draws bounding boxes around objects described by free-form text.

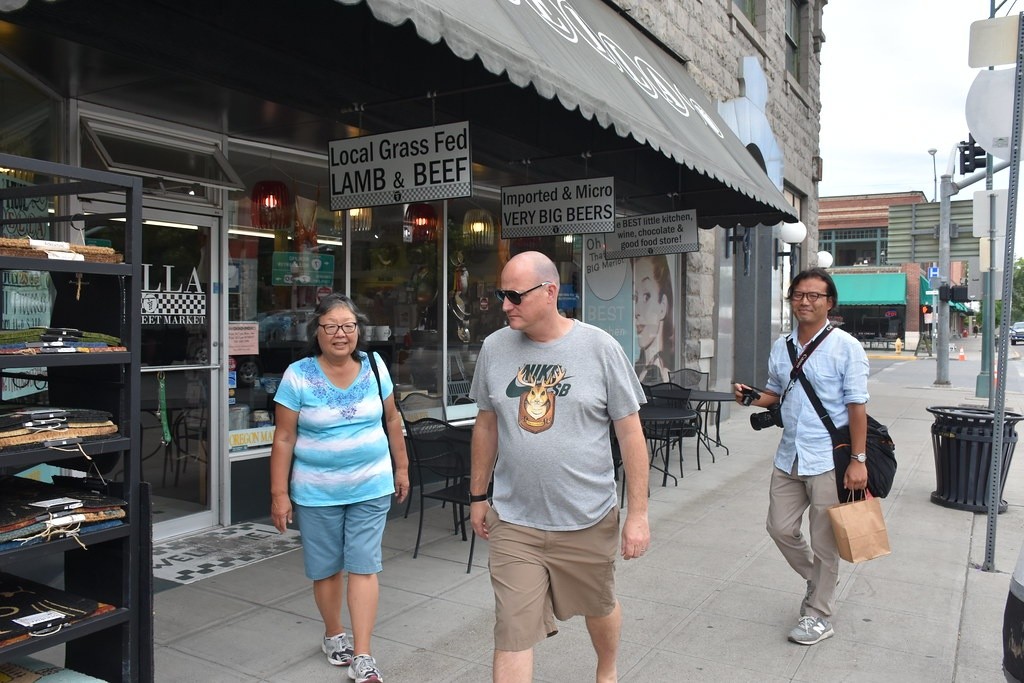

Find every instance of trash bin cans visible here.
[925,406,1023,514]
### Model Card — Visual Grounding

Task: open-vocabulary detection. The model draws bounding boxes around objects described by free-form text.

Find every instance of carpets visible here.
[153,521,303,593]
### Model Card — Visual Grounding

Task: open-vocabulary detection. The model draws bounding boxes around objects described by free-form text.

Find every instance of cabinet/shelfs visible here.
[0,150,145,683]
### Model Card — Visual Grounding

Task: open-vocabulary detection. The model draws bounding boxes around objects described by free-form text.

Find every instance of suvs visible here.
[1009,322,1024,346]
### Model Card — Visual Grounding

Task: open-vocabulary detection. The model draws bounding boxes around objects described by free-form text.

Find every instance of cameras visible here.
[751,403,784,431]
[741,389,761,406]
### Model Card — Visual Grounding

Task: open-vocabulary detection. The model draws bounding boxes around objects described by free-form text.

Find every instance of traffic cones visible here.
[958,347,966,362]
[993,359,997,387]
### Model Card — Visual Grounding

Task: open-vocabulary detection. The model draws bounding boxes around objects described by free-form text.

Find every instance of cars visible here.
[229,308,395,390]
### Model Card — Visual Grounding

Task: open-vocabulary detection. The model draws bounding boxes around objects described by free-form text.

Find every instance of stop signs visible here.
[316,286,332,304]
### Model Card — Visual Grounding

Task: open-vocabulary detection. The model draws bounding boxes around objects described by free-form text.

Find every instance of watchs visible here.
[468,493,488,503]
[851,453,867,463]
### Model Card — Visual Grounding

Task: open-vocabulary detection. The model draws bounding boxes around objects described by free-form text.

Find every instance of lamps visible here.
[404,202,439,242]
[462,207,494,249]
[817,251,833,268]
[334,207,372,233]
[250,179,291,230]
[775,220,808,269]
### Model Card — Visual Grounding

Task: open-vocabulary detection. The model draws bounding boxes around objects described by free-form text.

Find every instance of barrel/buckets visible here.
[407,328,440,365]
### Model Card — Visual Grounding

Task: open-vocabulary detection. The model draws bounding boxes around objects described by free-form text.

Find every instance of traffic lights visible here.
[921,304,932,314]
[958,140,975,175]
[938,284,951,303]
[967,132,987,173]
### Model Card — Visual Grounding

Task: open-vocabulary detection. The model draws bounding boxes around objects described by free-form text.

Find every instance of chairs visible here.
[638,368,730,487]
[395,392,470,535]
[403,417,494,574]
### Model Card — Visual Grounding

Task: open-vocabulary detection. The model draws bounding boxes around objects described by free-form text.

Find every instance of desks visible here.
[140,397,206,485]
[639,406,697,487]
[443,423,500,541]
[644,389,738,463]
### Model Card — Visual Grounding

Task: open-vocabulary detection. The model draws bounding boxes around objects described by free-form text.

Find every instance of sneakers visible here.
[789,614,834,645]
[347,654,383,683]
[320,631,354,665]
[800,585,814,617]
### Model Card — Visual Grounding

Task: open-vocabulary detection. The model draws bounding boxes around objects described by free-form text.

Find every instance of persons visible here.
[973,325,978,338]
[270,293,409,683]
[631,256,675,386]
[470,250,651,683]
[732,268,870,645]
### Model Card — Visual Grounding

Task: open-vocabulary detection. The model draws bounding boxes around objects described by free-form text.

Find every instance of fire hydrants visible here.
[893,337,902,354]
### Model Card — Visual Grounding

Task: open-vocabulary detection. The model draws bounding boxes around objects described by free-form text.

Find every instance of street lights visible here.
[927,147,938,202]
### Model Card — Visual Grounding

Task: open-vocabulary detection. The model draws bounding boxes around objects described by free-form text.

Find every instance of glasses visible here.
[496,282,551,304]
[317,322,358,335]
[791,291,828,302]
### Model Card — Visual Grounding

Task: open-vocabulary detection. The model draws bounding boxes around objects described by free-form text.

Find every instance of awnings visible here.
[830,274,907,305]
[920,275,954,307]
[344,0,800,229]
[950,301,968,312]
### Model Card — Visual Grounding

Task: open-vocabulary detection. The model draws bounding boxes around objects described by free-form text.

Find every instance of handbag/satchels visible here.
[826,486,891,564]
[831,413,898,501]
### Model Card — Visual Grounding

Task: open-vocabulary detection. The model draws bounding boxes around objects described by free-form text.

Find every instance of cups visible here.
[376,326,391,341]
[363,326,376,341]
[253,410,270,421]
[277,322,295,341]
[265,377,281,394]
[296,322,308,342]
